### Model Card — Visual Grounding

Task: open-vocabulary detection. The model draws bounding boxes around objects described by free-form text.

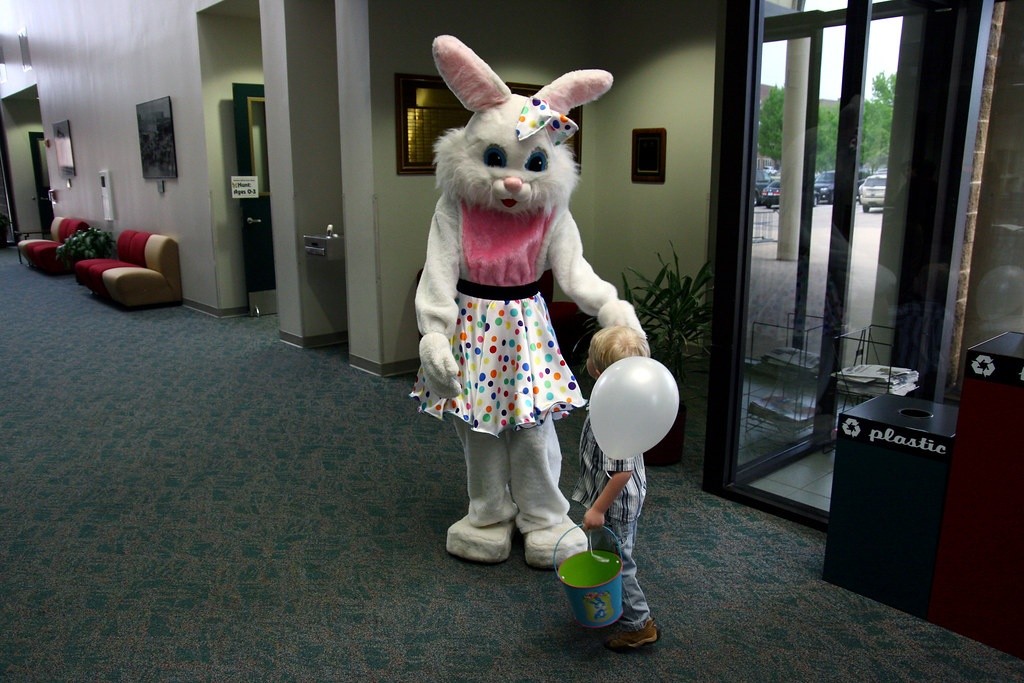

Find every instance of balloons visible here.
[589,355,679,461]
[975,265,1024,319]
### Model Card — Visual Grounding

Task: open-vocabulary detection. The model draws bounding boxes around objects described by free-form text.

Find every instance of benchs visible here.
[416,268,591,362]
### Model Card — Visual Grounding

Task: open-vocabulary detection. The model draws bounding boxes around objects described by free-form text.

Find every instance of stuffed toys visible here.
[409,35,648,567]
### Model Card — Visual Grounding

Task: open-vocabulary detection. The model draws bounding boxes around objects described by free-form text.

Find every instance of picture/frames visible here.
[631,127,667,184]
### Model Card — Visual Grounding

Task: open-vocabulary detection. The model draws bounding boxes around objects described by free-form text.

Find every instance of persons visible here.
[571,326,662,651]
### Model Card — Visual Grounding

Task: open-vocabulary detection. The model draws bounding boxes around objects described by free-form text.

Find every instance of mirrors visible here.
[393,72,583,175]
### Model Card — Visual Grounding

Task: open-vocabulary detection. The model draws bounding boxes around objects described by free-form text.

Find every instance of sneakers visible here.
[605,615,658,649]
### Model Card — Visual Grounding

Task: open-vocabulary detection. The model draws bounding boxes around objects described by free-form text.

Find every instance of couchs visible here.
[17,217,90,277]
[75,230,182,312]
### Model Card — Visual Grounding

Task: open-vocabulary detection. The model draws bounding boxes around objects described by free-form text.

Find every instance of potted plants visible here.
[57,225,117,285]
[0,213,13,249]
[573,241,715,465]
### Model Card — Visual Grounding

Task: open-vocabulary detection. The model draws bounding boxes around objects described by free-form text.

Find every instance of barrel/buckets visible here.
[553,523,625,628]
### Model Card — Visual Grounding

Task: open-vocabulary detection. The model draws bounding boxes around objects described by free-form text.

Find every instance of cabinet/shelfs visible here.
[743,311,824,447]
[821,322,929,455]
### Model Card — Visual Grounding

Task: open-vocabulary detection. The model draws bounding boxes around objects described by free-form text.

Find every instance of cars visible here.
[813,169,835,207]
[753,165,780,208]
[857,167,887,212]
